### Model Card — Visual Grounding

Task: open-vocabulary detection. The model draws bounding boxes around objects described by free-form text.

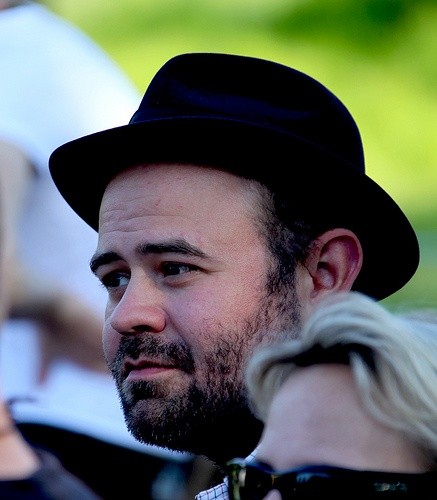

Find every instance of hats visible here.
[48,52,420,300]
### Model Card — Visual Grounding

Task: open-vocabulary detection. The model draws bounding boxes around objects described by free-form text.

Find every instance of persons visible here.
[0,53,437,500]
[0,0,194,500]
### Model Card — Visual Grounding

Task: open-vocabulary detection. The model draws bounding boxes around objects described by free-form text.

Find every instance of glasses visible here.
[228,458,437,500]
